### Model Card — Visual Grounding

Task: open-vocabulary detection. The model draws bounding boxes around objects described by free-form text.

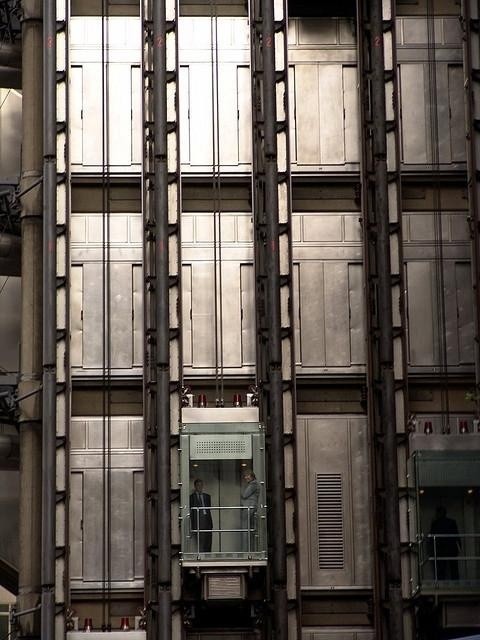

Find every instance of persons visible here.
[429,508,464,581]
[239,469,261,551]
[190,478,213,553]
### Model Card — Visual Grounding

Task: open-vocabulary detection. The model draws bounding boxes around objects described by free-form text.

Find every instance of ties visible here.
[199,493,202,507]
